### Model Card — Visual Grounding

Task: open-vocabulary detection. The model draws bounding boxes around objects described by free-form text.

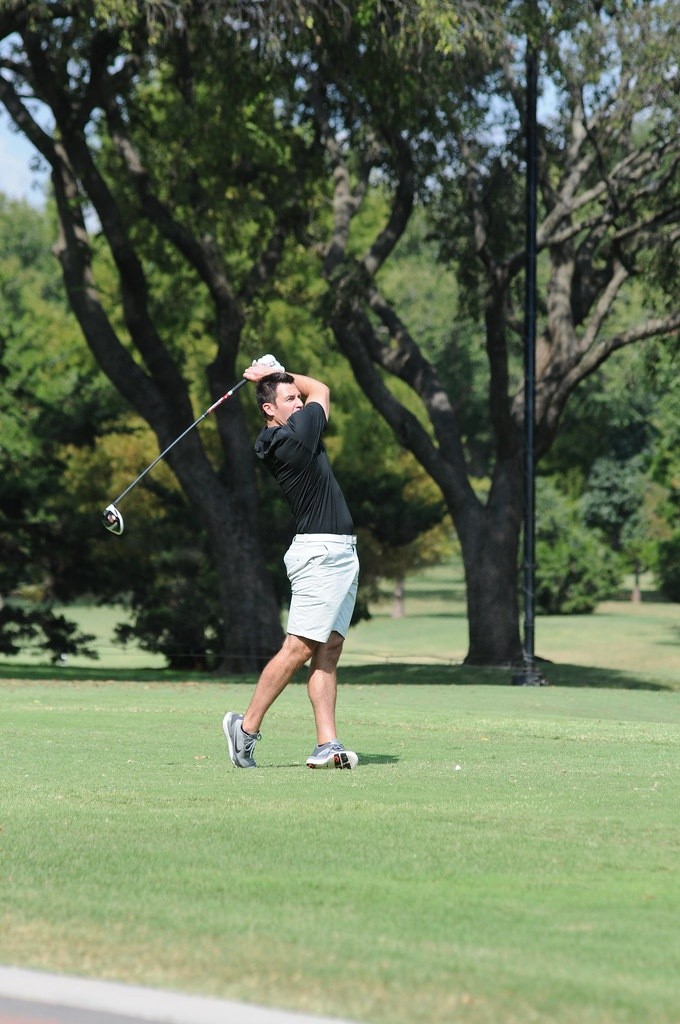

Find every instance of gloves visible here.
[251,354,286,374]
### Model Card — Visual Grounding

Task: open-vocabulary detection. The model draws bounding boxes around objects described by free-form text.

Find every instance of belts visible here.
[294,533,358,545]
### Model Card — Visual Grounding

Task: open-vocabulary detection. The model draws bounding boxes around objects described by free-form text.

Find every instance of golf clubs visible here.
[102,377,249,536]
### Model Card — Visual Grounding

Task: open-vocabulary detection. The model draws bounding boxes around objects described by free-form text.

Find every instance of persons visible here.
[222,354,360,769]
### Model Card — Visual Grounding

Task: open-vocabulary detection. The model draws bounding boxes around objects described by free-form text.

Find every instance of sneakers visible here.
[305,739,359,771]
[222,711,262,769]
